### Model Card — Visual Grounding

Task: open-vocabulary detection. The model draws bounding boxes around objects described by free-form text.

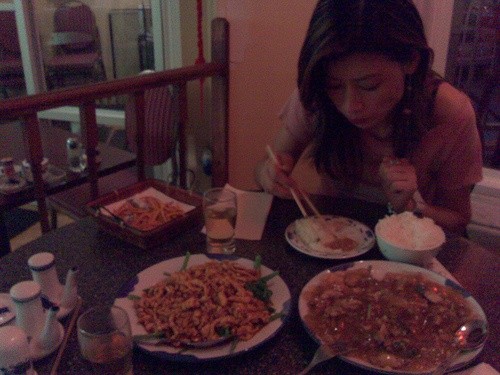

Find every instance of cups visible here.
[22,158,50,186]
[202,188,237,255]
[77,305,135,375]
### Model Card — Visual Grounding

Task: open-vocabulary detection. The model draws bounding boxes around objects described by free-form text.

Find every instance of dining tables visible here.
[0,191,500,375]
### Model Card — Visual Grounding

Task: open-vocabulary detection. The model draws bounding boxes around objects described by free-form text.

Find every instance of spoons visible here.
[439,319,489,373]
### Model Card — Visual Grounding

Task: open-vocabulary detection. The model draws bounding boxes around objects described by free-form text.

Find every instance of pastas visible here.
[112,196,184,230]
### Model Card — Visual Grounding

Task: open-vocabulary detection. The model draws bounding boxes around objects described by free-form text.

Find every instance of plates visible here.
[285,214,376,258]
[0,292,17,326]
[298,259,489,373]
[113,254,291,361]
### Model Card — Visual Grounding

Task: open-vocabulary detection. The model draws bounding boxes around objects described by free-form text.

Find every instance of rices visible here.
[377,210,444,249]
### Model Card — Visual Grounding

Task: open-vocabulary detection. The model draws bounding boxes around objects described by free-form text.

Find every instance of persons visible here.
[253,1,482,232]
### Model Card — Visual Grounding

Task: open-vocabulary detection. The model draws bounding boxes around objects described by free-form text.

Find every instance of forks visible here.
[291,340,358,375]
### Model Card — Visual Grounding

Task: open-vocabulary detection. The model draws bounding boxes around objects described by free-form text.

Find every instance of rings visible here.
[390,159,401,166]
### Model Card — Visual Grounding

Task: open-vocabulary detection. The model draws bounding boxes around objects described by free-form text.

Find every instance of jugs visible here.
[10,281,65,360]
[28,252,83,319]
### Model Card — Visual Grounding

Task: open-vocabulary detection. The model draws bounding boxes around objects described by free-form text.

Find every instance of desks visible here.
[1,117,136,257]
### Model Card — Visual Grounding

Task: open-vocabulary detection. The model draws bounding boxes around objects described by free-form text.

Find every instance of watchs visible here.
[411,190,426,215]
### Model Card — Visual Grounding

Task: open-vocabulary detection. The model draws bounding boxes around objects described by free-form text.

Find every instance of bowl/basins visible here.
[376,217,446,266]
[85,180,203,250]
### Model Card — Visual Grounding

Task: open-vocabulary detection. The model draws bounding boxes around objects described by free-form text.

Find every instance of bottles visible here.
[68,137,103,173]
[0,326,41,375]
[1,157,16,184]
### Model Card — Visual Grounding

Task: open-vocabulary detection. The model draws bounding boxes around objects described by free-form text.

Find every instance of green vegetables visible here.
[126,251,286,354]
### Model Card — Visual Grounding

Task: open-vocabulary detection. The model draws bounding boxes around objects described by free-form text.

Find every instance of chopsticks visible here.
[265,145,338,241]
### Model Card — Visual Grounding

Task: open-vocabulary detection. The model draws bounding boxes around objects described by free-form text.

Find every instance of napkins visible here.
[201,184,272,240]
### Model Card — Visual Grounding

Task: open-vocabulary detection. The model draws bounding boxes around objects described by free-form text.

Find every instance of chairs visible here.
[0,11,26,100]
[43,0,107,91]
[47,84,180,233]
